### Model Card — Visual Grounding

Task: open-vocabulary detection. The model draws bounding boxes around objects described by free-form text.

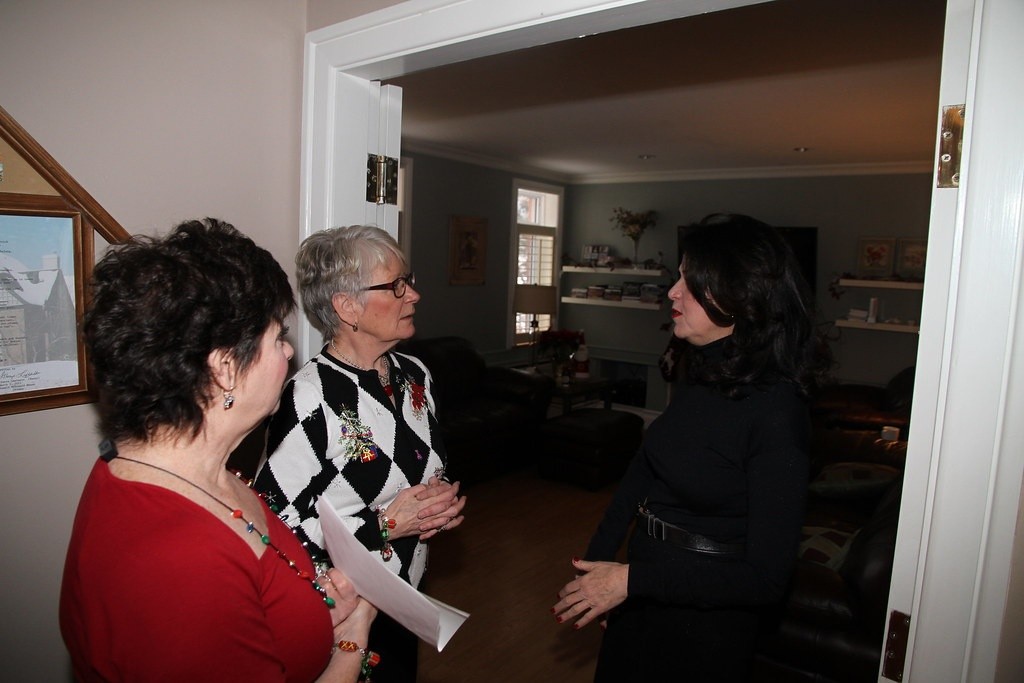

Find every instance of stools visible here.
[536,407,645,491]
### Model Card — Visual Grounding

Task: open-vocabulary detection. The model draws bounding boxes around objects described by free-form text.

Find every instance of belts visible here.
[637,507,744,555]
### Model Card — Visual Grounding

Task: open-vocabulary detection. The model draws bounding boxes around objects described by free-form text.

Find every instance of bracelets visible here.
[331,642,380,683]
[378,507,396,563]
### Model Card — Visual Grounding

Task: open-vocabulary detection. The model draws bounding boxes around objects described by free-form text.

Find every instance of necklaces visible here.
[114,455,335,610]
[328,337,392,394]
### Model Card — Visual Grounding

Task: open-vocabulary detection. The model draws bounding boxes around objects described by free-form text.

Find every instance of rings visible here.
[439,526,446,532]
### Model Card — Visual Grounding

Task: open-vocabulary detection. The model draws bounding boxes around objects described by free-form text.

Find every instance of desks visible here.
[517,365,612,410]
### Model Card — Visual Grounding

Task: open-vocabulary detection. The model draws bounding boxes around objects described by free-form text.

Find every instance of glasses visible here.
[360,270,416,298]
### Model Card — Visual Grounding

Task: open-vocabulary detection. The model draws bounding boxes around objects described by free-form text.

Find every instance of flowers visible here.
[535,326,585,367]
[609,203,655,241]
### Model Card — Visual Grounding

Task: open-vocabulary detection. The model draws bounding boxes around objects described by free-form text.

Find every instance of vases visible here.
[630,238,641,266]
[549,356,569,388]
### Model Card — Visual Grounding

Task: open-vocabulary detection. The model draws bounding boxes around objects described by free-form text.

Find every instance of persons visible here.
[238,223,468,683]
[548,212,832,682]
[57,219,377,683]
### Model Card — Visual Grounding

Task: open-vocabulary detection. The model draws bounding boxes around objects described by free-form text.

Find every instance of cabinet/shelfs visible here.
[833,277,924,334]
[561,265,671,311]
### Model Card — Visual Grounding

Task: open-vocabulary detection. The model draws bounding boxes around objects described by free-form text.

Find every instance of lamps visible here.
[513,282,557,343]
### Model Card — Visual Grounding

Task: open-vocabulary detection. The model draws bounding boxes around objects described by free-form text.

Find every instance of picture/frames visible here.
[856,235,897,278]
[896,236,927,279]
[0,190,94,402]
[447,208,488,285]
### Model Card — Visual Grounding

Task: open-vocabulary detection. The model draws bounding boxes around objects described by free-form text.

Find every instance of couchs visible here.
[747,425,908,683]
[398,335,549,491]
